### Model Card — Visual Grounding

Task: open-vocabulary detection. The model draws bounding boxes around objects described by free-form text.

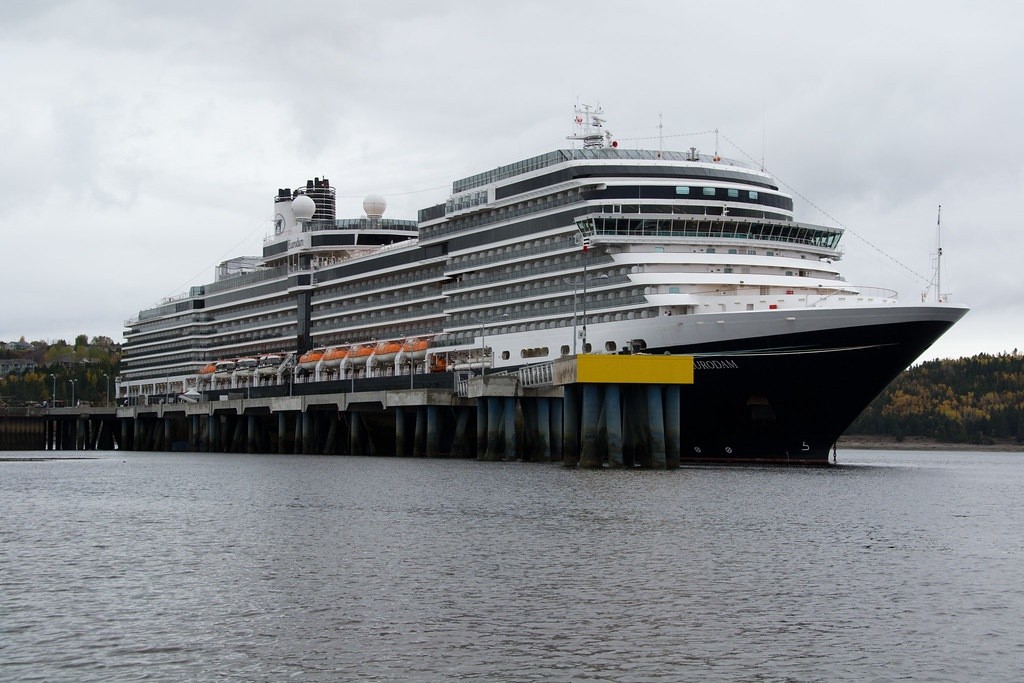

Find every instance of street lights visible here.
[474,312,510,376]
[563,274,611,353]
[46,372,171,405]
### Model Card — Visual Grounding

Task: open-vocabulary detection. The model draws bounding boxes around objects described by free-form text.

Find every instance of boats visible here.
[421,353,493,373]
[298,338,424,371]
[201,351,282,383]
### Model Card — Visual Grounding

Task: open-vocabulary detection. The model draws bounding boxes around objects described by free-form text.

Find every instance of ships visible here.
[111,99,970,474]
